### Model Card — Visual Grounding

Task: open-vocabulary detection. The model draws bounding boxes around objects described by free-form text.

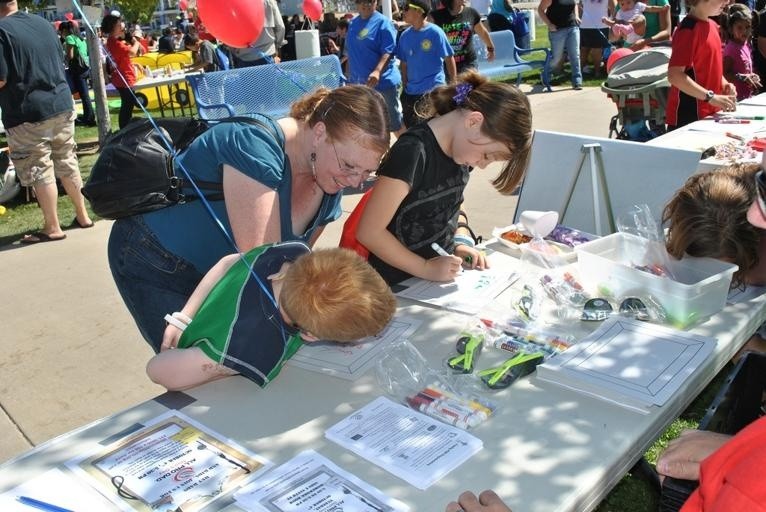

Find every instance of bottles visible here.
[144,60,187,80]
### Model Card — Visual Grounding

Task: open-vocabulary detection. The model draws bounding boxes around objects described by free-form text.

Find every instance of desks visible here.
[125,63,203,120]
[632,91,766,178]
[0,215,766,510]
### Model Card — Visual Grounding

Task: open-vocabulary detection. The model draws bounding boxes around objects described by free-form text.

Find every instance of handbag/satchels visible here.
[83,117,207,222]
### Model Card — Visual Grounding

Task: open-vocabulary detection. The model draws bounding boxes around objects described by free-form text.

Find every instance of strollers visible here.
[602,43,679,143]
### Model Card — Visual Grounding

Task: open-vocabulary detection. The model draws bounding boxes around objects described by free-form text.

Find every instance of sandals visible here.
[19,226,71,246]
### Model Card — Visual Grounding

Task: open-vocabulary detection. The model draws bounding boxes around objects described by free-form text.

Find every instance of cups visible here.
[521,210,559,239]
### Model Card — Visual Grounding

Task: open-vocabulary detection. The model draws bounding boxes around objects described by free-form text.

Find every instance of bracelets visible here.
[168,311,193,328]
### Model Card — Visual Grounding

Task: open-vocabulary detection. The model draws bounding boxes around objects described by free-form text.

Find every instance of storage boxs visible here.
[572,231,741,331]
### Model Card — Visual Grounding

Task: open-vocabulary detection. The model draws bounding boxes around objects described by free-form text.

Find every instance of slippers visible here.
[72,216,94,228]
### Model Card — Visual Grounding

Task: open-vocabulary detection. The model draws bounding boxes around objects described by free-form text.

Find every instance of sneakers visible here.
[594,70,601,79]
[574,84,583,90]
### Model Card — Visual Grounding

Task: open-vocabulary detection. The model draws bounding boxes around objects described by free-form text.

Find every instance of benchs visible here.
[469,29,553,93]
[185,54,349,127]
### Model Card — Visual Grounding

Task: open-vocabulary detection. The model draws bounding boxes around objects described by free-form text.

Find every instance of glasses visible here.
[479,349,547,388]
[580,300,653,324]
[330,135,379,185]
[447,331,485,375]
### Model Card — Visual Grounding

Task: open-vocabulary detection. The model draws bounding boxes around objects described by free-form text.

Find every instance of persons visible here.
[0,0,94,244]
[352,64,535,305]
[144,238,399,392]
[104,82,390,379]
[440,411,766,511]
[653,162,765,288]
[0,0,766,126]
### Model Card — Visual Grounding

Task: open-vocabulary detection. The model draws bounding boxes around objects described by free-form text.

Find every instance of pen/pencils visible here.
[476,318,571,363]
[541,275,566,304]
[405,380,496,430]
[715,114,765,124]
[564,272,590,298]
[430,242,464,272]
[15,495,75,512]
[726,131,744,140]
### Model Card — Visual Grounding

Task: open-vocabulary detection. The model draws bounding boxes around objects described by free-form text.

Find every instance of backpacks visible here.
[338,187,374,263]
